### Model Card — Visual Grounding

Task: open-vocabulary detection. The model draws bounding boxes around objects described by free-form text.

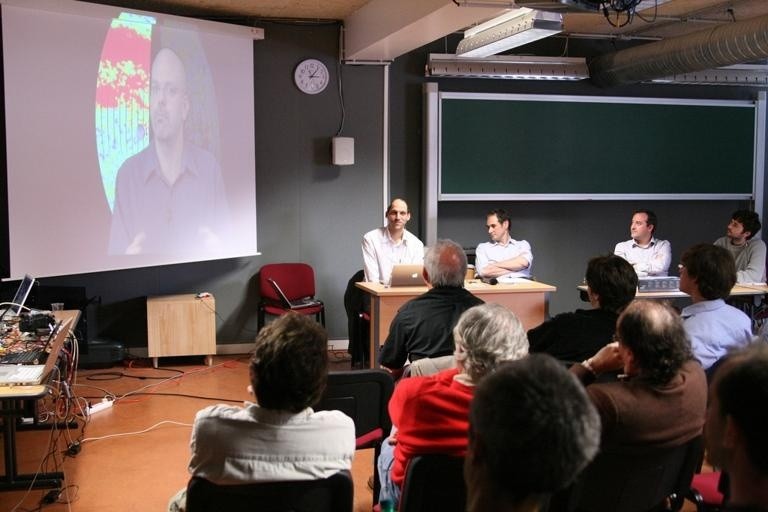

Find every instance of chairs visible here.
[256,264,326,331]
[465,264,474,280]
[344,271,370,368]
[186,352,729,512]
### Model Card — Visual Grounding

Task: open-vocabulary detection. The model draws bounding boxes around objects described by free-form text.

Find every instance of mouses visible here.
[198,291,211,298]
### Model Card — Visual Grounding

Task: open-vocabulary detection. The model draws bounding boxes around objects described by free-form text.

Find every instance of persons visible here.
[367,240,485,490]
[476,209,533,279]
[362,198,424,282]
[379,302,528,511]
[465,353,602,511]
[713,211,767,332]
[527,255,638,368]
[679,244,752,369]
[107,45,226,256]
[570,300,708,512]
[169,310,357,512]
[710,347,767,511]
[614,209,671,276]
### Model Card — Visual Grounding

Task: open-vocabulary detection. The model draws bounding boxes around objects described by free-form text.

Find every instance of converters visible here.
[43,490,61,503]
[69,444,81,454]
[21,417,34,423]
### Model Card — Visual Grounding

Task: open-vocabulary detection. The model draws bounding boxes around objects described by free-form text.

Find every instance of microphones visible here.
[481,277,497,285]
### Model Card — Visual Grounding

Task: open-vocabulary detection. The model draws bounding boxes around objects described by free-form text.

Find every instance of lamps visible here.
[455,6,563,60]
[423,34,590,81]
[640,63,768,89]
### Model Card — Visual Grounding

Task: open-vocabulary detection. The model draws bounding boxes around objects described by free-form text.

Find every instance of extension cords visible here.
[84,400,114,415]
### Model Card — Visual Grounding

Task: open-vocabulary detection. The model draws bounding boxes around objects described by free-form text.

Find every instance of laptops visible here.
[0,320,63,364]
[267,278,321,309]
[0,273,35,317]
[380,263,426,287]
[0,315,74,385]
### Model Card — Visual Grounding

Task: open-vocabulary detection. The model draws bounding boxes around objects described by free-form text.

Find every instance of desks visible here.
[577,284,768,332]
[0,309,82,485]
[146,294,216,366]
[356,280,557,369]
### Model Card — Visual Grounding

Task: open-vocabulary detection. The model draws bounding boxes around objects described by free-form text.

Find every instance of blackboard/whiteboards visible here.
[422,82,766,201]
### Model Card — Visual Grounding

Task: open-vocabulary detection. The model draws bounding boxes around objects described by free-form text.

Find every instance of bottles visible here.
[379,498,395,512]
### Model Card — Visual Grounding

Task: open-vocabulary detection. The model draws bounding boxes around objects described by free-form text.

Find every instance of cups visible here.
[50,303,64,319]
[464,264,475,281]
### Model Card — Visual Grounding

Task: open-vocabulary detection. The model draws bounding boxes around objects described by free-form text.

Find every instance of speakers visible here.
[331,136,356,166]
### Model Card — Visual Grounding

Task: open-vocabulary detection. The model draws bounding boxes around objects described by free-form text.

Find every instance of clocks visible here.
[292,59,330,95]
